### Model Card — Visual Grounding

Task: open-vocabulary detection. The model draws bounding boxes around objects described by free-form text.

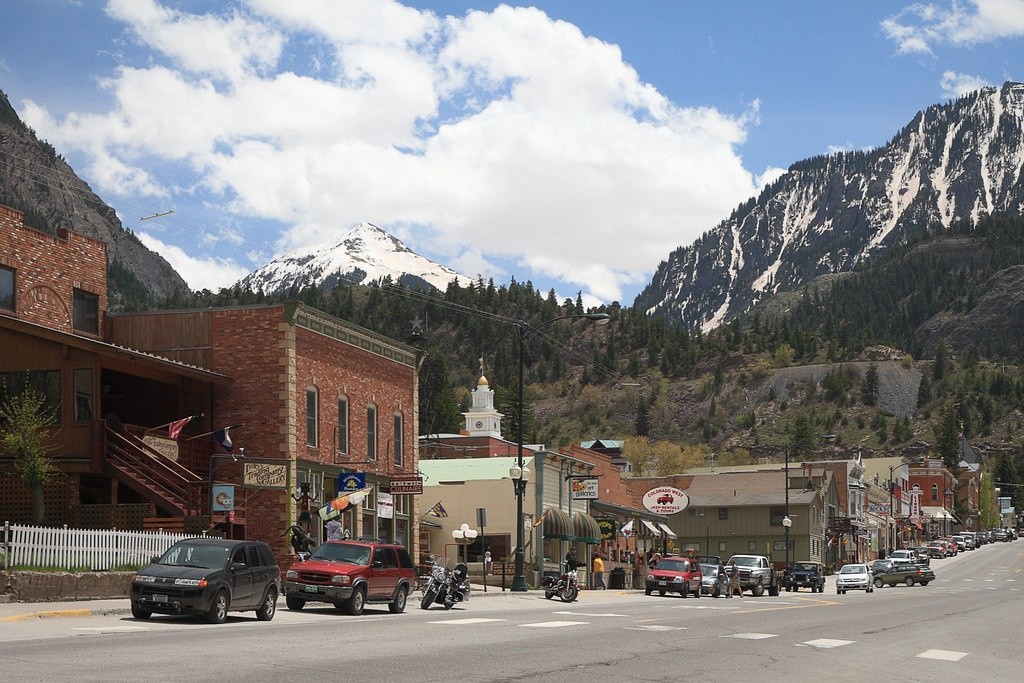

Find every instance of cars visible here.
[785,561,826,593]
[129,538,281,624]
[927,528,1024,560]
[693,563,734,598]
[887,550,918,565]
[835,564,874,595]
[874,564,936,589]
[907,547,931,566]
[870,560,894,575]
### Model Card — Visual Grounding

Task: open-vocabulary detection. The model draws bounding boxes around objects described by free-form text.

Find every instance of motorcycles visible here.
[541,560,580,603]
[421,553,470,610]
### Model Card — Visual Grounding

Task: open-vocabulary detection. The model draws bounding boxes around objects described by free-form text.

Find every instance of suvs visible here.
[645,556,703,598]
[285,537,416,615]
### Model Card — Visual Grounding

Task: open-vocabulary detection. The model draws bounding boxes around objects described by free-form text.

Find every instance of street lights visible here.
[783,435,837,576]
[943,466,969,536]
[977,478,1001,531]
[452,524,477,587]
[889,462,925,556]
[509,313,611,593]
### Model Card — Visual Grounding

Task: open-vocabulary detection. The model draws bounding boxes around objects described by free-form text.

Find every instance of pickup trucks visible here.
[724,554,784,597]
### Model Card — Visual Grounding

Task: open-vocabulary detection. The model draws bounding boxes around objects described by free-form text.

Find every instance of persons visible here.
[594,552,606,590]
[728,562,743,598]
[649,553,661,566]
[565,546,578,571]
[1008,529,1012,542]
[485,551,491,574]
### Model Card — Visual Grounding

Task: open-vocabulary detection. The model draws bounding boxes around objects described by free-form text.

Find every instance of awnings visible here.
[544,507,575,542]
[642,521,661,536]
[658,524,677,539]
[573,512,600,546]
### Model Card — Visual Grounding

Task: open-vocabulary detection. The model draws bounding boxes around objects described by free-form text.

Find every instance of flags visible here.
[429,502,448,518]
[169,415,197,438]
[621,521,632,538]
[214,427,235,452]
[824,537,834,552]
